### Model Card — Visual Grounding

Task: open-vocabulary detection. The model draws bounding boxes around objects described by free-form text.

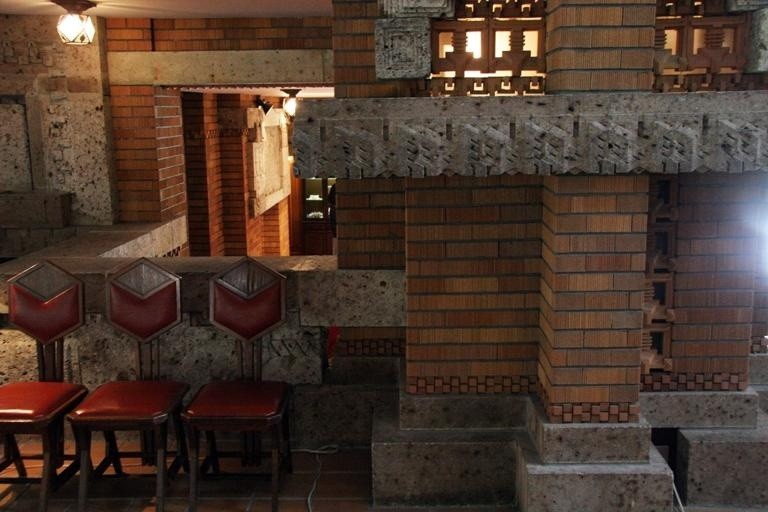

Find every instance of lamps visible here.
[50,0,99,47]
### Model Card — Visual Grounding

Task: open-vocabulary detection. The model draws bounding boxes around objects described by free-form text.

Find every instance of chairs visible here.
[66,258,192,510]
[180,258,292,510]
[3,257,89,509]
